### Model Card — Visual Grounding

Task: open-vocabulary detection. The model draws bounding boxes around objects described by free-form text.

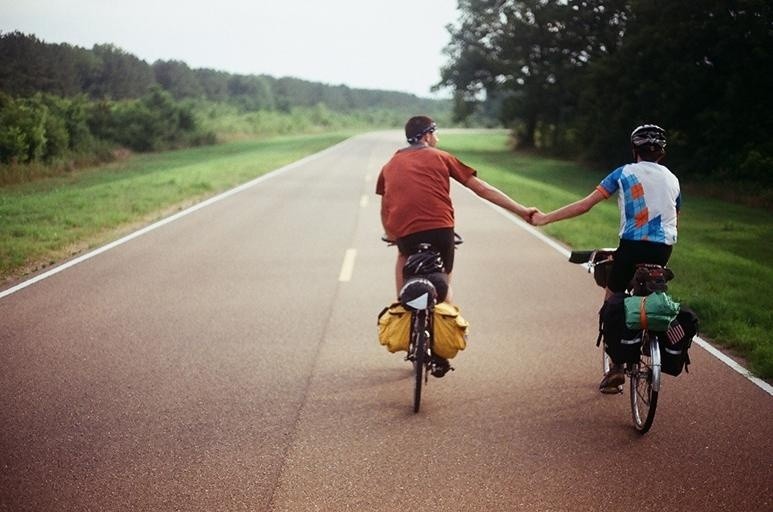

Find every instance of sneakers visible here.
[598,370,625,395]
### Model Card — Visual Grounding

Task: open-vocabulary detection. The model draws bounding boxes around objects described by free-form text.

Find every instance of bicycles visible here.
[380,233,468,416]
[564,240,672,434]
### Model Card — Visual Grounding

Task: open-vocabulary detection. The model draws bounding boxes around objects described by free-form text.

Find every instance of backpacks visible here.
[375,301,413,355]
[594,291,645,378]
[660,306,699,377]
[431,300,470,360]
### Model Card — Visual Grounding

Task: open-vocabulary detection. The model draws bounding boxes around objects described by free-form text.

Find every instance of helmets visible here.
[629,123,667,152]
[400,247,446,281]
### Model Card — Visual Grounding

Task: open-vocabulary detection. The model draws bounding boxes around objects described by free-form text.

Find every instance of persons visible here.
[375,115,542,374]
[531,125,682,390]
